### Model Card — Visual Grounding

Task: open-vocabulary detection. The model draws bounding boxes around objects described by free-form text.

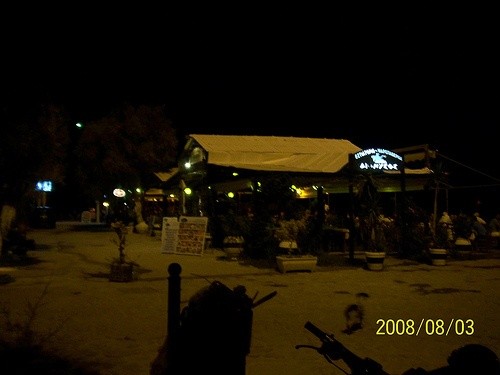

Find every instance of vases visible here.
[456,242,472,259]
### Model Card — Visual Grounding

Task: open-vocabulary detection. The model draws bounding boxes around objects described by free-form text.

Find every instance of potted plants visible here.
[355,213,395,270]
[222,235,245,260]
[266,209,319,273]
[109,221,142,282]
[430,229,448,266]
